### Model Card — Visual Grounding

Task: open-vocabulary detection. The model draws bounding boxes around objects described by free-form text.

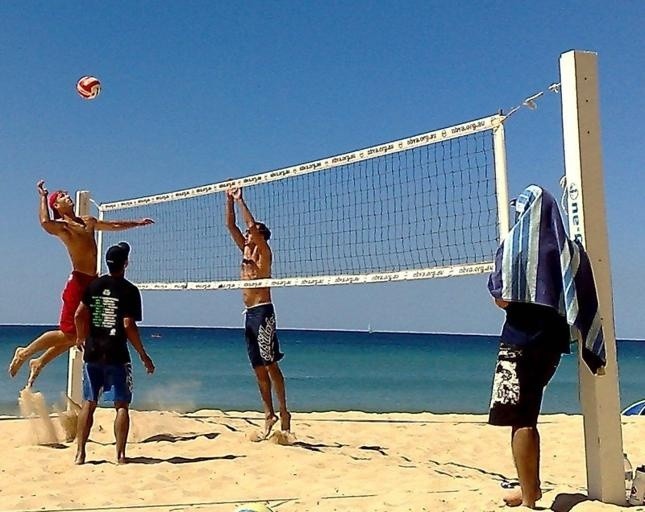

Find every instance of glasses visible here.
[246,229,265,234]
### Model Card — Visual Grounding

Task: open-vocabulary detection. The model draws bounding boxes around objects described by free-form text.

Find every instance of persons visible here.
[9,179,155,388]
[487,187,579,507]
[223,188,291,440]
[73,240,155,464]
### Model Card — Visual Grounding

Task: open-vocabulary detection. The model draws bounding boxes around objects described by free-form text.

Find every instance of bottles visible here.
[623,453,645,506]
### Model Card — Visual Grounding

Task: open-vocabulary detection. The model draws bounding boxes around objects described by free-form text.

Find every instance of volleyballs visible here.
[76,76,101,100]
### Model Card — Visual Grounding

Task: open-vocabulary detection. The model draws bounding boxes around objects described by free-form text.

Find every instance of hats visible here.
[48,190,65,220]
[105,241,130,267]
